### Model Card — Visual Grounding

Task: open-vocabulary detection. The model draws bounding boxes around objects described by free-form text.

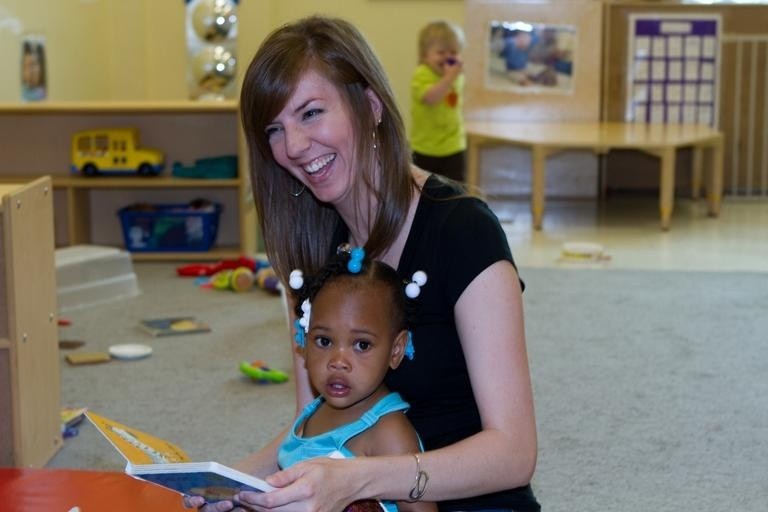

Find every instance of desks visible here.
[0,462,217,512]
[463,121,727,231]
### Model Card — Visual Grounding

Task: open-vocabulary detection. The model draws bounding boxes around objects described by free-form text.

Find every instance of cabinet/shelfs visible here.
[0,100,263,264]
[0,175,64,472]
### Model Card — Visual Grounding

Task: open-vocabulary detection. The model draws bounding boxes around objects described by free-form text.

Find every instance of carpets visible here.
[43,264,768,512]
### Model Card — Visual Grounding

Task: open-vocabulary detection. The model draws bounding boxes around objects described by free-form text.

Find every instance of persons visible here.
[180,13,540,512]
[276,246,440,512]
[497,27,562,87]
[411,19,467,181]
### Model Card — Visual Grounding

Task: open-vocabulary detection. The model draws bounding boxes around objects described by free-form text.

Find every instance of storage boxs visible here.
[55,244,143,315]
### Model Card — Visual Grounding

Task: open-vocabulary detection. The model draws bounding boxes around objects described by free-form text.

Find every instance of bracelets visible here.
[406,452,427,503]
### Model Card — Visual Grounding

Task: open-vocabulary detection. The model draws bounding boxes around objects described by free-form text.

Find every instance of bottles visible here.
[19,41,47,102]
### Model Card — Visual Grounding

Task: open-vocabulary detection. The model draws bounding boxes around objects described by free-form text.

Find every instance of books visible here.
[139,314,210,337]
[84,408,277,505]
[59,403,85,435]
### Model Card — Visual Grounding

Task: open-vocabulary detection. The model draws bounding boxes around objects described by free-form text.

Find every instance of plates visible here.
[109,344,152,360]
